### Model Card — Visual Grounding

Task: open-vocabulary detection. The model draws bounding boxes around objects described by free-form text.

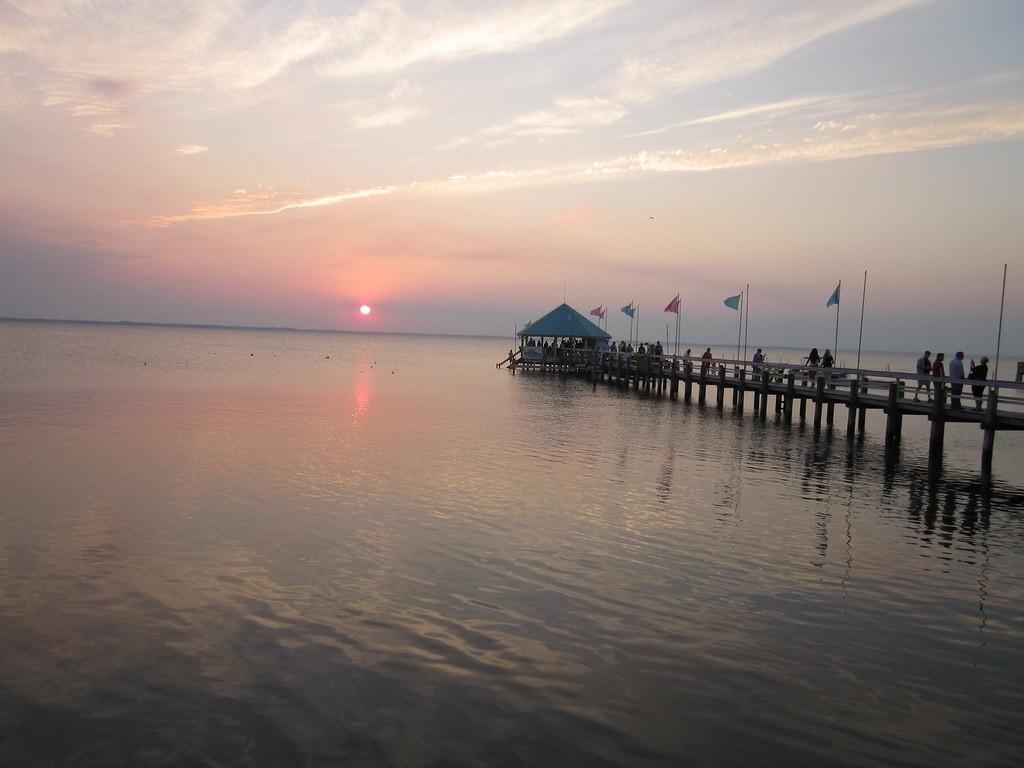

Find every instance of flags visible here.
[724,295,741,311]
[589,305,602,316]
[597,309,607,320]
[620,303,632,315]
[662,296,682,316]
[826,285,840,307]
[624,306,637,319]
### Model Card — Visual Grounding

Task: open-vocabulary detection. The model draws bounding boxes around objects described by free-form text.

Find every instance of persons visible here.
[930,352,948,405]
[701,347,712,378]
[526,334,672,381]
[819,348,835,389]
[682,348,692,373]
[913,350,935,403]
[804,348,821,390]
[947,350,966,410]
[710,359,717,376]
[969,355,989,411]
[752,347,767,379]
